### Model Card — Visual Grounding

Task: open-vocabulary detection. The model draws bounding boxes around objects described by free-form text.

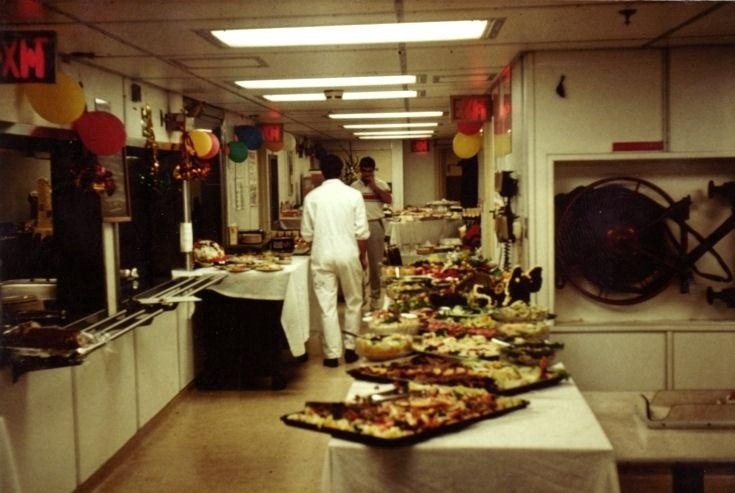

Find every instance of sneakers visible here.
[370,297,380,310]
[322,358,338,367]
[344,350,357,363]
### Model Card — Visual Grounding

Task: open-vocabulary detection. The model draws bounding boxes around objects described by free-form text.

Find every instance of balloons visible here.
[452,133,482,159]
[75,111,126,156]
[457,98,486,135]
[25,72,84,124]
[201,132,219,159]
[185,130,213,157]
[234,125,263,150]
[228,141,248,163]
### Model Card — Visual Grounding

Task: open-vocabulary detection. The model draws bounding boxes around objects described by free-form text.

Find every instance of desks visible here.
[321,294,619,491]
[192,255,313,391]
[230,208,314,254]
[382,214,462,252]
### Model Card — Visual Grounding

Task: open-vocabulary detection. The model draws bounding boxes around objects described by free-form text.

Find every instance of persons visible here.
[349,157,392,311]
[300,154,371,368]
